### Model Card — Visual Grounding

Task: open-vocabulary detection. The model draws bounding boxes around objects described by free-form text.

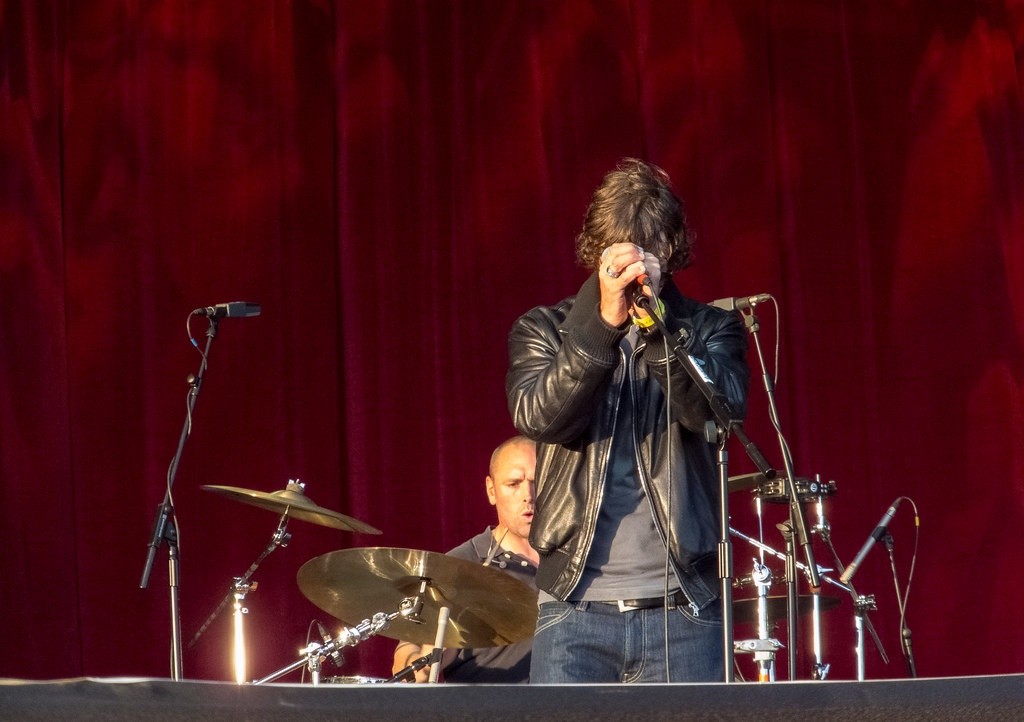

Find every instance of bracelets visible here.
[630,298,665,328]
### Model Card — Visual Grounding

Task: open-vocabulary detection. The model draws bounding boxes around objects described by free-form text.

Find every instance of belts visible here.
[600,591,689,613]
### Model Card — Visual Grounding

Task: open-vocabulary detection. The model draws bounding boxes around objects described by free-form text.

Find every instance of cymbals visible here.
[731,594,839,623]
[197,484,379,535]
[296,546,539,649]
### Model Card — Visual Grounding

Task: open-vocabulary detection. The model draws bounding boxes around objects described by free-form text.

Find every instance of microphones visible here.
[192,301,261,319]
[429,607,450,684]
[317,622,345,667]
[706,294,773,312]
[637,272,652,287]
[840,498,902,584]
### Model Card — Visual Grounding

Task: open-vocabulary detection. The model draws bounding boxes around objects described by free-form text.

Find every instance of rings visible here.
[606,266,620,278]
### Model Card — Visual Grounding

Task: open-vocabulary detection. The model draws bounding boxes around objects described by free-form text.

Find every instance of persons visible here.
[506,157,748,684]
[393,435,537,685]
